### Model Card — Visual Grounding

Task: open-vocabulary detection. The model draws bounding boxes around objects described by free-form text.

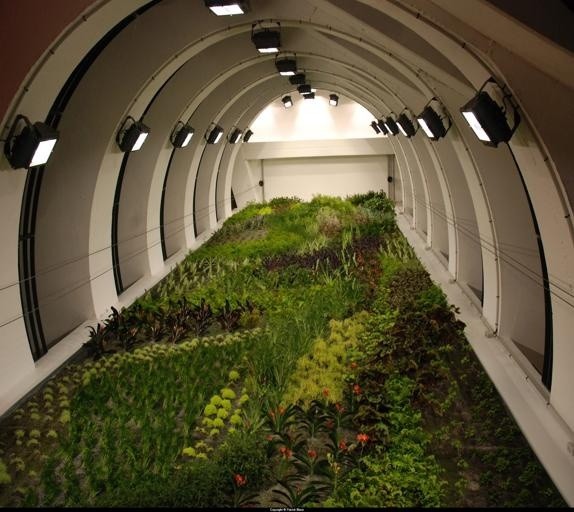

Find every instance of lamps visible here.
[360,77,521,149]
[0,114,255,169]
[329,94,338,106]
[206,1,315,108]
